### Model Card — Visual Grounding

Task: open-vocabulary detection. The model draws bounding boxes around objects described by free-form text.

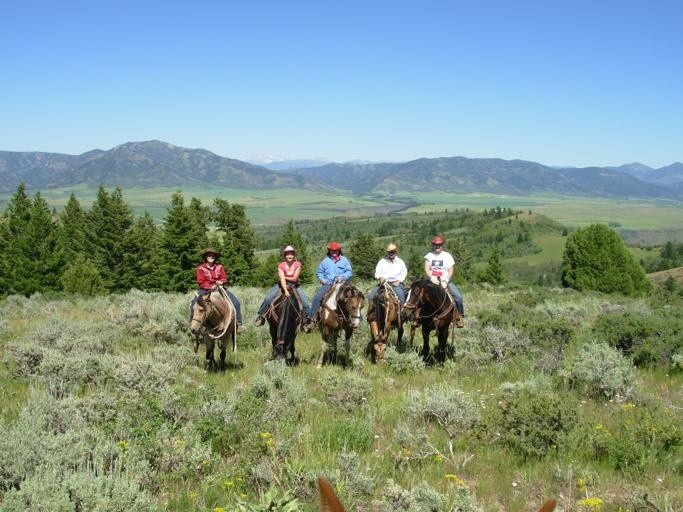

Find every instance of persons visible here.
[367,242,407,307]
[253,244,314,333]
[423,235,464,328]
[307,241,364,334]
[186,247,247,336]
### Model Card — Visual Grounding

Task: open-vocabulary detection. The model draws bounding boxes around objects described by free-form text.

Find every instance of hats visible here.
[200,247,220,262]
[281,245,297,257]
[327,241,341,250]
[432,235,443,245]
[383,242,397,253]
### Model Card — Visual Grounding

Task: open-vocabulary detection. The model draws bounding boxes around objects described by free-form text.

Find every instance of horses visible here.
[366,286,404,364]
[316,280,365,370]
[267,284,300,365]
[189,289,235,373]
[400,276,454,368]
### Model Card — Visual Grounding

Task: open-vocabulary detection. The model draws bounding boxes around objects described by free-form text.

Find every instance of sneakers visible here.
[236,325,246,333]
[456,318,464,328]
[254,315,265,326]
[302,321,316,333]
